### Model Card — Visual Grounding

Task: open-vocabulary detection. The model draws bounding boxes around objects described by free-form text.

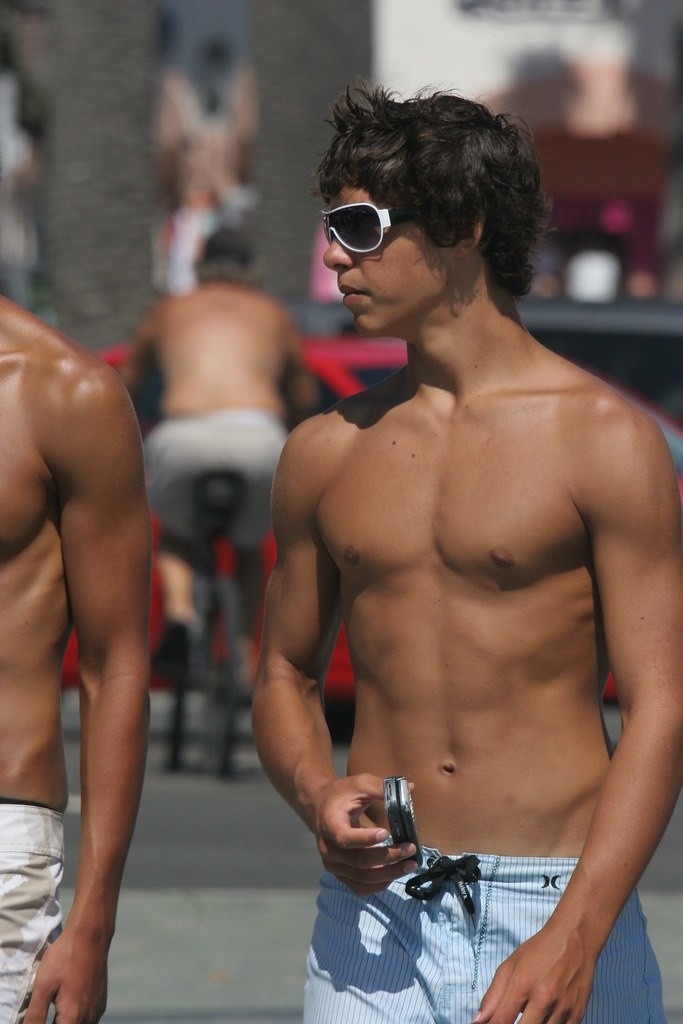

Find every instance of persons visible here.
[0,292,153,1024]
[118,228,319,683]
[153,34,264,293]
[250,95,683,1024]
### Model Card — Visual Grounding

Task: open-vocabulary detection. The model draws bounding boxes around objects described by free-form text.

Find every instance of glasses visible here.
[320,202,428,253]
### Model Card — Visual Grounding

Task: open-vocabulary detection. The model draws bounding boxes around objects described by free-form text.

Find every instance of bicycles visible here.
[169,472,248,780]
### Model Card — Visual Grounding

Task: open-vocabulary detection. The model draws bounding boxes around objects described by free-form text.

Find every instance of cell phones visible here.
[382,775,422,866]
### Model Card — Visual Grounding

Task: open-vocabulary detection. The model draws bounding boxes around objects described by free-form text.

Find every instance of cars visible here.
[59,335,682,714]
